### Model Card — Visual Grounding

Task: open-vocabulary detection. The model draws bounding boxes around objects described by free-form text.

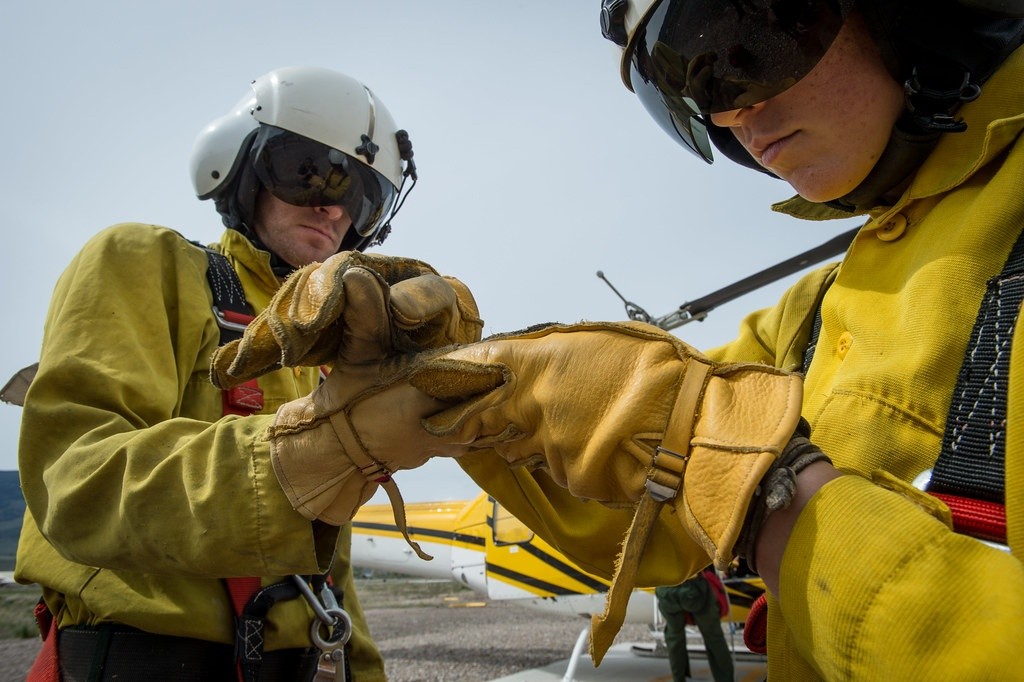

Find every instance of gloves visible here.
[267,264,497,527]
[208,249,485,394]
[408,320,805,571]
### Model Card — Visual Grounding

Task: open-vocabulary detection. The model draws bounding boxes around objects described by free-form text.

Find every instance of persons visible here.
[211,0,1024,682]
[655,560,736,682]
[0,69,470,682]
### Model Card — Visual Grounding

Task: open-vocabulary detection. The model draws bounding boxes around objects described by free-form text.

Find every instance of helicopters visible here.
[350,222,871,653]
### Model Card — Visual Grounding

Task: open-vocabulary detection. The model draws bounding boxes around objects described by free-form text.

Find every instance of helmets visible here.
[597,0,1024,217]
[189,63,418,256]
